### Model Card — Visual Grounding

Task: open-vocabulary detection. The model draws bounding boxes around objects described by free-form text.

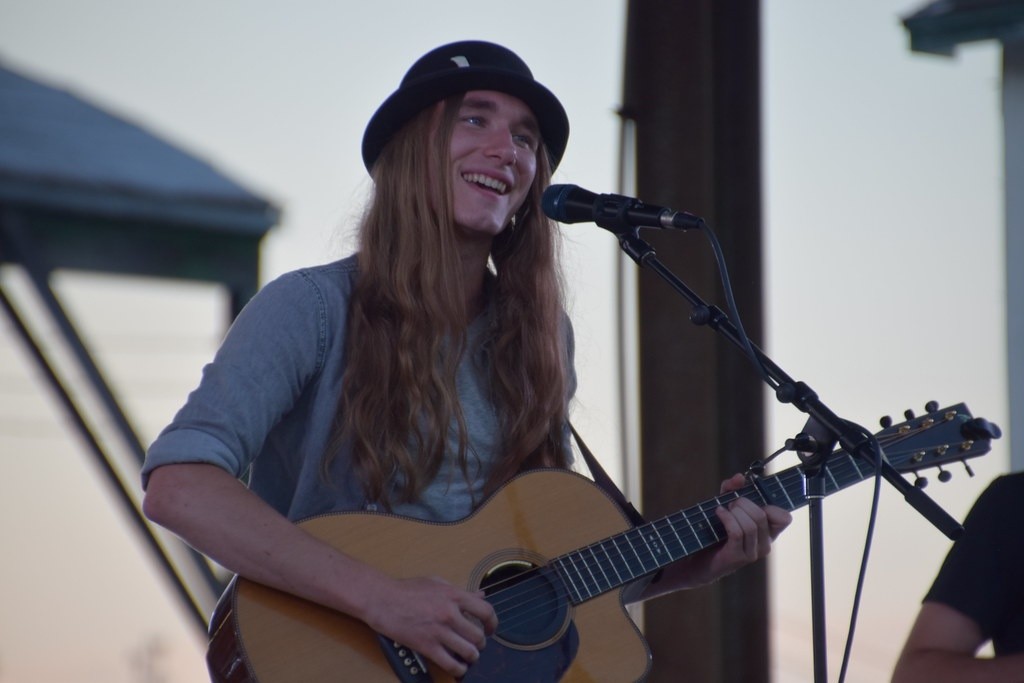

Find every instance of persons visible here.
[141,36,795,676]
[890,470,1024,683]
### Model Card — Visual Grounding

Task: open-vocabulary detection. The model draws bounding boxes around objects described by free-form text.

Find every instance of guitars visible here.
[208,398,1003,682]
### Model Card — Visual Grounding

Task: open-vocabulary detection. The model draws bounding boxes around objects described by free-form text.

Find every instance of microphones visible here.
[541,185,706,231]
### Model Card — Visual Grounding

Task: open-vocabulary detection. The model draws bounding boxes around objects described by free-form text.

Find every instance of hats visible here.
[360,40,569,187]
